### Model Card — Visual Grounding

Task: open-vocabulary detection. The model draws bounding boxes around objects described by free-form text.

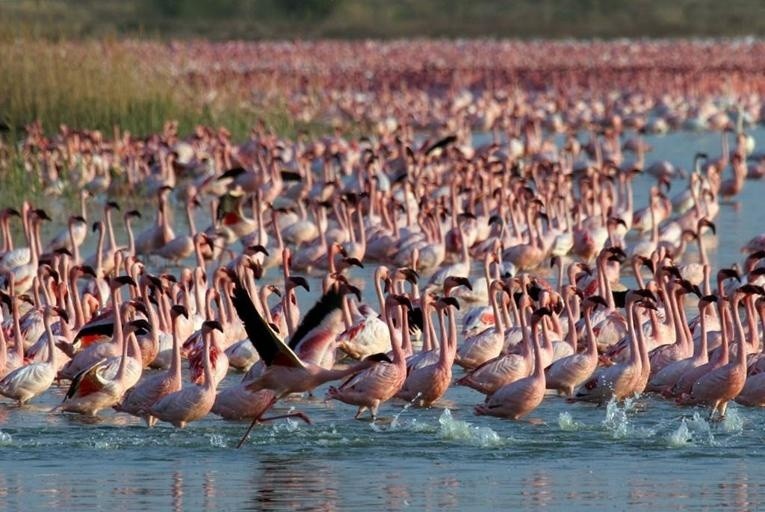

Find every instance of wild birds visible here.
[0,27,765,452]
